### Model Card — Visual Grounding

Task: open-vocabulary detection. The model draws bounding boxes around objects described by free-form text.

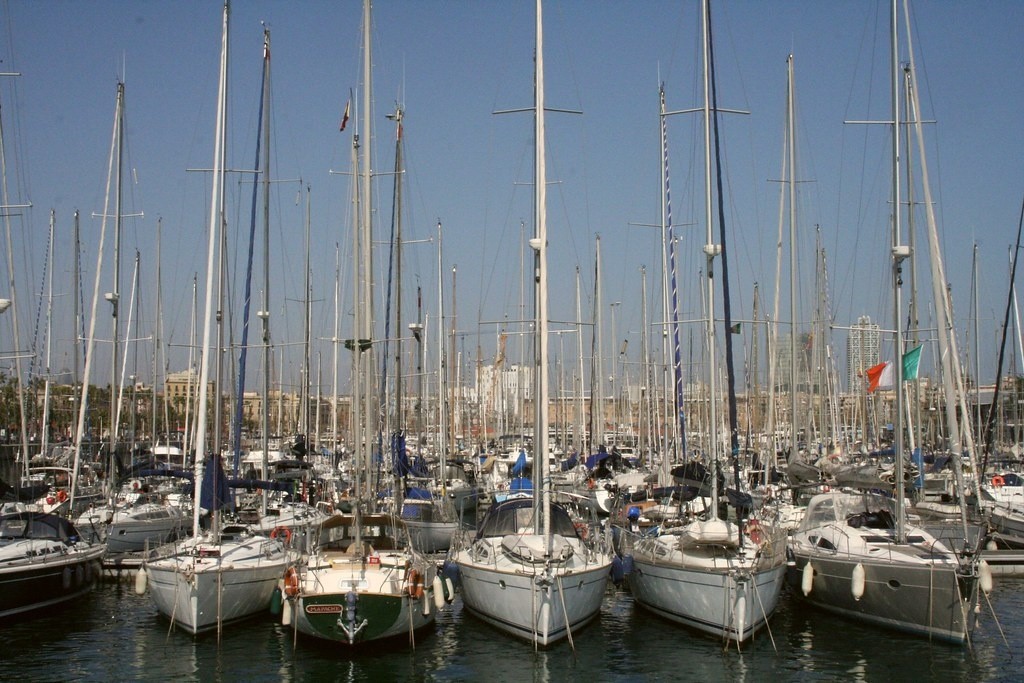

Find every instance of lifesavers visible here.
[301,490,307,501]
[589,478,593,488]
[270,526,290,546]
[409,569,424,600]
[256,488,262,495]
[283,567,297,595]
[824,483,831,492]
[575,523,587,538]
[130,480,141,491]
[992,475,1004,487]
[56,490,67,502]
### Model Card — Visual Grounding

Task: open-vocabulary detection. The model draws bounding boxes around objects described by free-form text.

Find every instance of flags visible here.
[866,358,895,393]
[901,343,925,381]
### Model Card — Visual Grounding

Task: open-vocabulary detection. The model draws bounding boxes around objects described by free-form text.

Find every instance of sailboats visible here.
[0,0,1024,662]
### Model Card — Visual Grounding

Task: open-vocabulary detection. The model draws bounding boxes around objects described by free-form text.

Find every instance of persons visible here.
[612,445,621,470]
[527,442,531,453]
[489,439,495,448]
[587,475,594,490]
